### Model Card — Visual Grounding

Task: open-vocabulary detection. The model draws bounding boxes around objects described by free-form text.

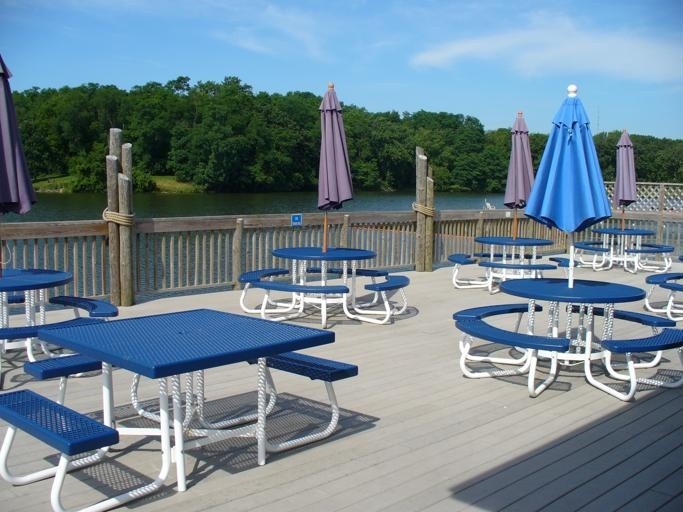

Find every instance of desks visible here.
[38,308,337,453]
[474,237,553,279]
[591,227,656,264]
[273,246,376,307]
[0,268,74,357]
[498,277,646,366]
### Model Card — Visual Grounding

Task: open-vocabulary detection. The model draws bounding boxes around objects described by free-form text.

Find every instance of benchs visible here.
[474,245,539,278]
[0,318,105,366]
[197,354,358,453]
[252,262,349,329]
[40,290,118,357]
[479,245,557,295]
[624,237,675,272]
[658,283,683,321]
[619,235,674,274]
[346,259,410,325]
[645,273,683,312]
[532,247,579,280]
[7,295,26,303]
[574,234,618,265]
[584,304,683,401]
[237,260,298,315]
[567,302,676,368]
[455,300,572,397]
[298,260,389,308]
[577,236,614,272]
[448,243,493,288]
[454,289,543,366]
[1,376,168,512]
[23,353,193,432]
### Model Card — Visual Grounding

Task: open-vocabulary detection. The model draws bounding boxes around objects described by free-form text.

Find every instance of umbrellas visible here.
[505,111,535,240]
[614,127,637,231]
[524,85,612,289]
[0,51,39,216]
[317,82,354,253]
